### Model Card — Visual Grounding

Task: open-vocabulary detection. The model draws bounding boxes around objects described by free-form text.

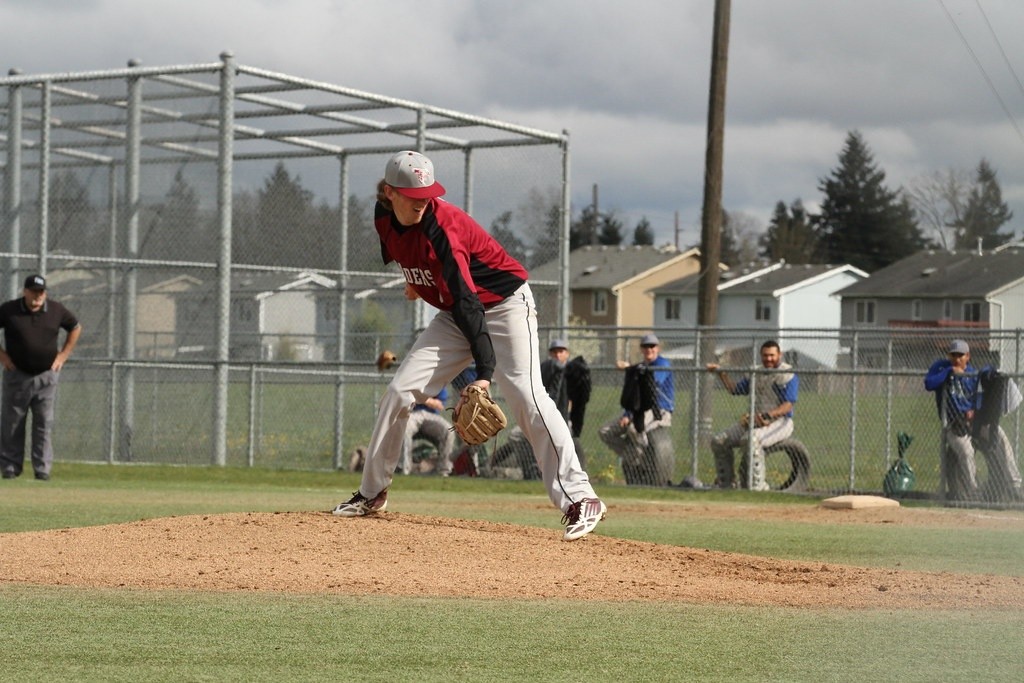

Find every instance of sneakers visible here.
[560,498,607,542]
[332,487,388,517]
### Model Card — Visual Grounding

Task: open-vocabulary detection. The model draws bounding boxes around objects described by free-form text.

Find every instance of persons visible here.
[507,339,592,476]
[0,274,82,480]
[924,339,1024,502]
[706,341,798,491]
[401,388,455,477]
[451,364,492,476]
[328,150,607,541]
[599,334,674,466]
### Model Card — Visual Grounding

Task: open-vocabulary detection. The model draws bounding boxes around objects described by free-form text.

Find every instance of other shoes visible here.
[35,473,49,480]
[2,468,14,478]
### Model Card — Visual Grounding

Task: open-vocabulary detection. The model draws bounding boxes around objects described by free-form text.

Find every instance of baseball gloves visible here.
[377,351,396,372]
[740,411,769,429]
[450,384,507,446]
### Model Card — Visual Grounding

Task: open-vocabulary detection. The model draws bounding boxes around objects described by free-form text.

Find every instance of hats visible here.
[385,150,446,199]
[548,339,568,351]
[948,340,969,354]
[640,335,659,345]
[24,275,46,292]
